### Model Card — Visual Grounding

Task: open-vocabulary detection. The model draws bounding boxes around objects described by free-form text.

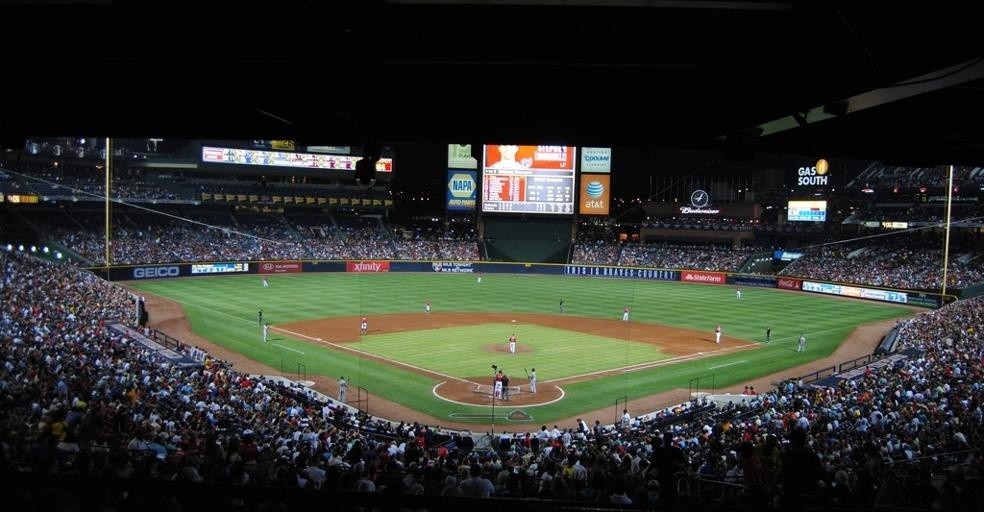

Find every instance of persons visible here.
[714,325,721,343]
[1,136,479,265]
[477,272,482,284]
[736,286,740,299]
[571,137,983,290]
[854,293,984,386]
[360,314,367,334]
[765,326,806,352]
[0,248,268,510]
[509,333,516,354]
[622,306,630,321]
[425,301,431,314]
[252,368,984,511]
[559,298,564,313]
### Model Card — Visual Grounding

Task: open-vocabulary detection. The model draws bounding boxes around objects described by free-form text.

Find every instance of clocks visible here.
[691,190,708,209]
